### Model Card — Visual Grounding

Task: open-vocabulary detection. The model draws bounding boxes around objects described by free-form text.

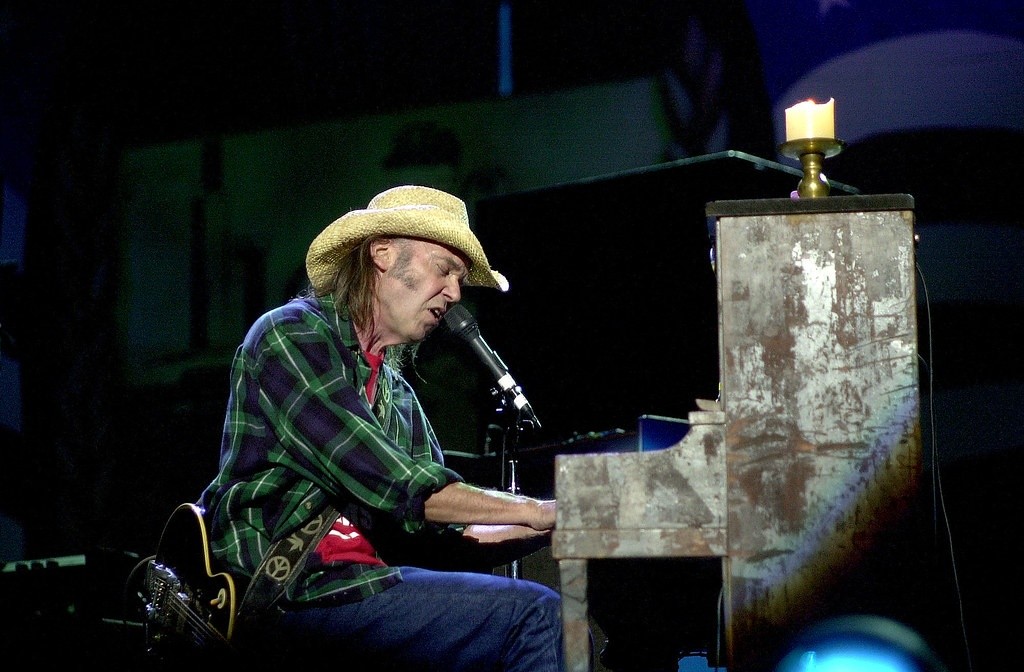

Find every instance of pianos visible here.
[553,176,924,670]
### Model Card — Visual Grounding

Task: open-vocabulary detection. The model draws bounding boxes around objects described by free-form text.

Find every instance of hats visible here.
[306,184,509,297]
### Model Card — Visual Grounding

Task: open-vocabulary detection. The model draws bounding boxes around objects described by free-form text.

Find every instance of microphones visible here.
[444,303,543,433]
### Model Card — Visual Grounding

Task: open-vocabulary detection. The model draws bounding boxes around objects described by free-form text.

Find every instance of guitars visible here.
[139,501,255,670]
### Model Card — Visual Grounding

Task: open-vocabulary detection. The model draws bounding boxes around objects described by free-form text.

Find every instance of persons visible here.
[193,185,595,672]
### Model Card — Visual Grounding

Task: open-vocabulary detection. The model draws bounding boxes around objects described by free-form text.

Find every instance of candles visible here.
[784,96,837,140]
